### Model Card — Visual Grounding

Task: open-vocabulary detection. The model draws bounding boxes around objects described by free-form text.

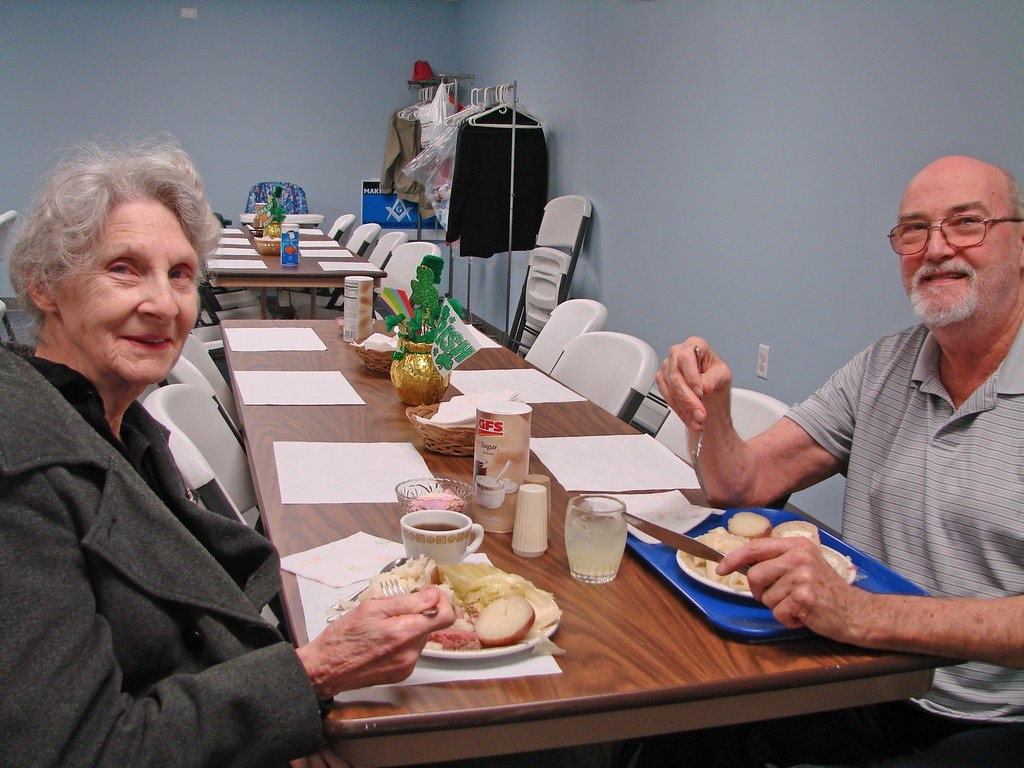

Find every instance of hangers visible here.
[398,80,543,128]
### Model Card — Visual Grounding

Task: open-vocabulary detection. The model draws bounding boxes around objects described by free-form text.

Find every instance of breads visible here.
[768,521,821,552]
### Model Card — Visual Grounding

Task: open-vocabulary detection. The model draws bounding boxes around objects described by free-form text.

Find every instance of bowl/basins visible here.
[335,317,376,337]
[394,478,474,519]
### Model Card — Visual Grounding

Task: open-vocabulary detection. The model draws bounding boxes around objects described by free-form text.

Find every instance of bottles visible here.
[511,484,549,558]
[524,474,553,543]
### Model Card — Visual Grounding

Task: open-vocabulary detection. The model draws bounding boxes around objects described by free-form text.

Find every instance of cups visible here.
[400,510,484,565]
[564,495,627,584]
[475,478,505,510]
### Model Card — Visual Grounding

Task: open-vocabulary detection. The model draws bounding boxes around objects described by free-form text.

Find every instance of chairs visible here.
[656,385,801,510]
[141,384,257,533]
[243,182,308,215]
[295,242,440,319]
[547,331,658,426]
[288,223,382,310]
[524,298,610,376]
[506,194,593,353]
[326,213,355,241]
[166,333,242,437]
[279,231,408,309]
[189,275,274,358]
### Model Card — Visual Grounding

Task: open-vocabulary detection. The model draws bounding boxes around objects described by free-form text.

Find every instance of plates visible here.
[416,581,561,659]
[675,532,856,596]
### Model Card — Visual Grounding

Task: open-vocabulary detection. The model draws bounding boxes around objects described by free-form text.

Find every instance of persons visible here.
[654,157,1024,768]
[0,126,459,768]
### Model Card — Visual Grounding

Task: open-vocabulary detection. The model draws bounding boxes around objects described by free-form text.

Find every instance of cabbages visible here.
[438,563,567,654]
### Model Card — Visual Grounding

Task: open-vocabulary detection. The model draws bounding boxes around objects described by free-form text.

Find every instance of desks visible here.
[203,225,388,318]
[221,315,970,768]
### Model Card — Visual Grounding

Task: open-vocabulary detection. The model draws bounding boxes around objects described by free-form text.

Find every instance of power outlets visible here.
[757,344,769,380]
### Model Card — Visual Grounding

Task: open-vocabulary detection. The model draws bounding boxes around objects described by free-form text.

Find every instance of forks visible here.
[691,346,711,501]
[380,578,438,616]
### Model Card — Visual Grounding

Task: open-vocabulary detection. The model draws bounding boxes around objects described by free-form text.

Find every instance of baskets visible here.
[254,237,280,255]
[405,402,476,456]
[352,339,396,374]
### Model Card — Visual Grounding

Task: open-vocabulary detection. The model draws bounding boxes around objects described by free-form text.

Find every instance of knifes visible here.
[621,512,749,577]
[331,558,409,610]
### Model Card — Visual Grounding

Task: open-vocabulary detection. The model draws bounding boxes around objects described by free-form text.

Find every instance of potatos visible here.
[727,512,772,539]
[473,596,536,645]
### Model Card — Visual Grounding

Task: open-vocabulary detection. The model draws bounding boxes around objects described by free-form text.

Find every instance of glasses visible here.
[886,214,1024,255]
